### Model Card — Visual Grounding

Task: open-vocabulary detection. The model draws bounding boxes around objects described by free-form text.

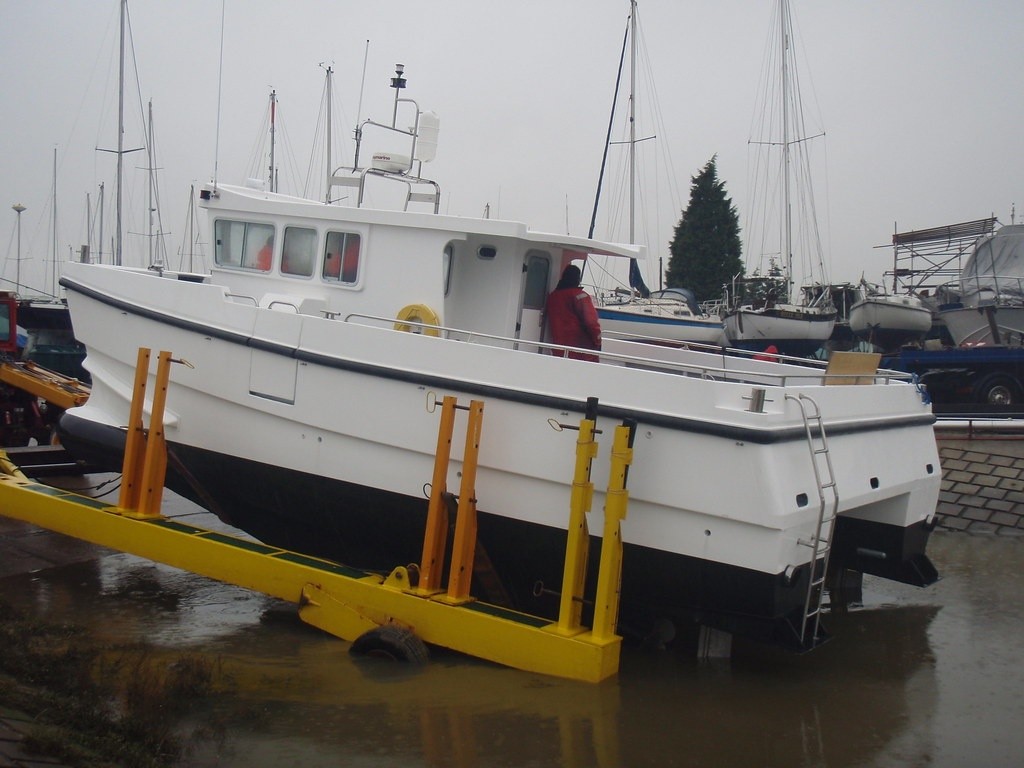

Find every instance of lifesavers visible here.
[392,304,441,338]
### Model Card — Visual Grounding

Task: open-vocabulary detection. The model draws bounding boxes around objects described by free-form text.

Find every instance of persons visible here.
[546,264,602,363]
[256,236,290,274]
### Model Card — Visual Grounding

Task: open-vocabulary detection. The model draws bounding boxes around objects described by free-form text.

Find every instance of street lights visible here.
[9,201,26,292]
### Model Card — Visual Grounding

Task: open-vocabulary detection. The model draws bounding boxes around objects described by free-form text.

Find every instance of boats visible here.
[3,0,948,685]
[915,213,1023,350]
[845,271,934,334]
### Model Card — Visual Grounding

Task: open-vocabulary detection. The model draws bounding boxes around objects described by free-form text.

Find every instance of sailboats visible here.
[577,0,729,352]
[720,0,838,342]
